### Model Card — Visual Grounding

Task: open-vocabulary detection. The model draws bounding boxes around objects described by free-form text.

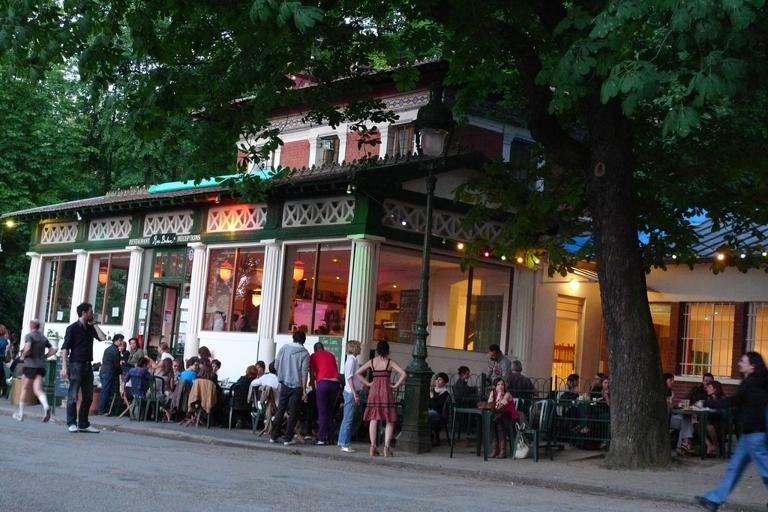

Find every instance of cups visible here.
[579,394,584,400]
[685,400,690,409]
[585,391,588,401]
[225,378,230,388]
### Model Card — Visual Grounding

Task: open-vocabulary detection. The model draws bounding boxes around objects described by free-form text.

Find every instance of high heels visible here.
[370,446,379,456]
[383,446,393,456]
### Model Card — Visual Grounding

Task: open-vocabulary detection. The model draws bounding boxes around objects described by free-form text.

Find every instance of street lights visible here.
[389,70,455,454]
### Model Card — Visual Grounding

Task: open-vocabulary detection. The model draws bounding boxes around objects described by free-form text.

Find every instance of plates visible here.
[680,409,692,411]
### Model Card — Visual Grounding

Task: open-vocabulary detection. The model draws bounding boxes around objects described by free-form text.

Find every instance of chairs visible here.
[259,368,734,459]
[0,362,265,428]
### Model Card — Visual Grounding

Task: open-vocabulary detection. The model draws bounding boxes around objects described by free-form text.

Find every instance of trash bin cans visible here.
[76,385,102,417]
[10,376,38,405]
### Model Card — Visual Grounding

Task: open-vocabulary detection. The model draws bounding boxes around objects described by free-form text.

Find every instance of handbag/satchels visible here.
[515,443,530,458]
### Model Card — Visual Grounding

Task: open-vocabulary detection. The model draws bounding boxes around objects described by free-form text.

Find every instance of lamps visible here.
[96,260,110,283]
[293,251,306,280]
[219,257,233,281]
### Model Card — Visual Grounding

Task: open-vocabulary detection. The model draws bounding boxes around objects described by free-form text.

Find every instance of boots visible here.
[488,439,506,459]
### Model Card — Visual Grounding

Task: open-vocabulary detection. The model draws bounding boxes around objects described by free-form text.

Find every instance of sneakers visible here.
[342,447,356,452]
[284,440,296,445]
[68,424,100,432]
[694,496,719,512]
[270,438,274,443]
